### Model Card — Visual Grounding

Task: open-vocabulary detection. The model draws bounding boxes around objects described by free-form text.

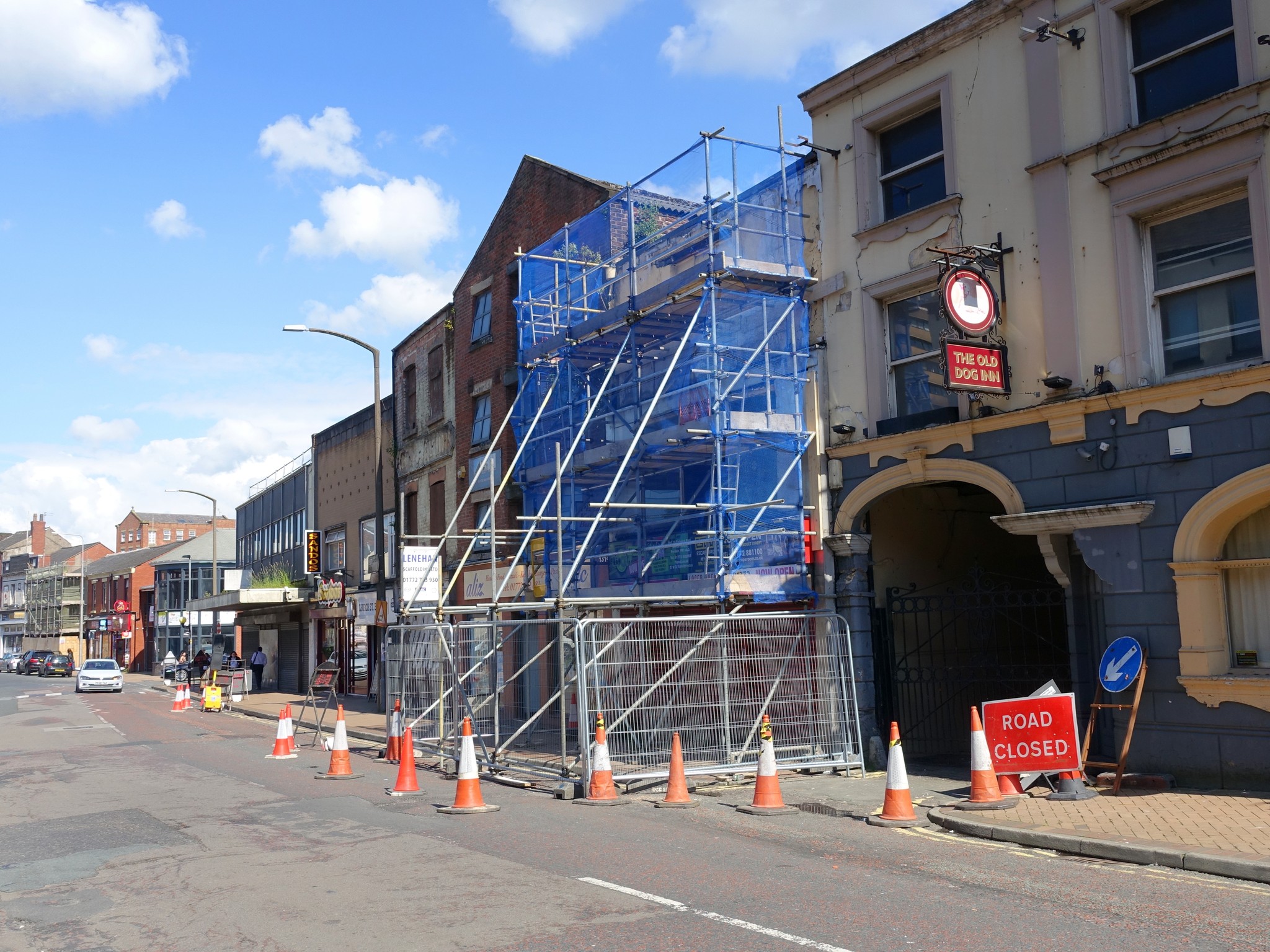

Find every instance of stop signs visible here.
[216,623,221,633]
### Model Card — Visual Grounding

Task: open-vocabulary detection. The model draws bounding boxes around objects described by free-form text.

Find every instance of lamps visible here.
[1041,376,1072,390]
[314,574,330,580]
[346,597,357,620]
[831,424,855,434]
[334,570,354,578]
[1020,17,1086,50]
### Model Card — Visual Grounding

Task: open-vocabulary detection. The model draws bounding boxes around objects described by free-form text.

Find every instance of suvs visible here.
[16,649,63,675]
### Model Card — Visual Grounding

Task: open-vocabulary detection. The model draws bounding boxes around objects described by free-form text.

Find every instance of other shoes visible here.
[256,687,261,690]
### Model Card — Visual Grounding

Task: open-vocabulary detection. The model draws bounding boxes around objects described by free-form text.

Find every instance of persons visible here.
[65,649,74,663]
[199,647,211,678]
[251,647,267,690]
[226,651,243,671]
[179,652,187,664]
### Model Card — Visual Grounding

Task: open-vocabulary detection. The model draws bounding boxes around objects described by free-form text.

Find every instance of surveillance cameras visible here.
[1077,448,1093,462]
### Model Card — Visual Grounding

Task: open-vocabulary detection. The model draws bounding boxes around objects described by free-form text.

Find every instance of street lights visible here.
[179,555,192,661]
[51,532,84,669]
[164,489,217,652]
[283,325,388,714]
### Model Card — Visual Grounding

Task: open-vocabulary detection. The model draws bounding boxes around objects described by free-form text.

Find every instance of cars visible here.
[74,659,125,693]
[38,654,73,678]
[201,644,213,658]
[0,653,25,673]
[160,653,231,682]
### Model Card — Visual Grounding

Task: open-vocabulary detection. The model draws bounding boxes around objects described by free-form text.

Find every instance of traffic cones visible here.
[373,699,401,764]
[654,732,700,808]
[263,704,301,760]
[572,713,632,806]
[385,727,428,797]
[736,715,801,815]
[868,722,931,827]
[200,684,206,706]
[169,684,193,712]
[954,706,1019,810]
[314,705,365,780]
[998,775,1034,799]
[1045,771,1099,801]
[436,717,501,814]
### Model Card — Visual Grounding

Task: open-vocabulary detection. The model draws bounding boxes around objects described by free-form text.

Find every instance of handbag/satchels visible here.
[250,663,254,672]
